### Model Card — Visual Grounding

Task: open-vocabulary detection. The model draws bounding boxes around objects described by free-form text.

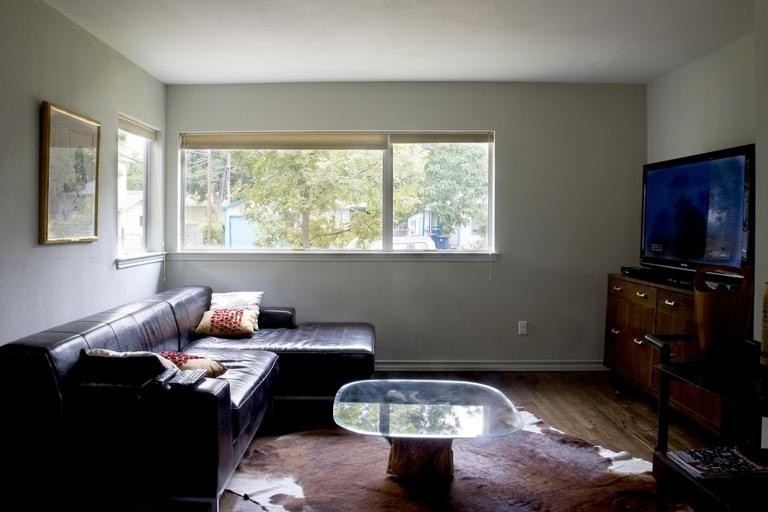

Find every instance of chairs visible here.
[643,333,768,454]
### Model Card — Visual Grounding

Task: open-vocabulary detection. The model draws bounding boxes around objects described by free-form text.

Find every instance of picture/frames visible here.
[40,101,103,245]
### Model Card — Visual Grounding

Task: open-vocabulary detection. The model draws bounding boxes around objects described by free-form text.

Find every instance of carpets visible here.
[225,404,692,512]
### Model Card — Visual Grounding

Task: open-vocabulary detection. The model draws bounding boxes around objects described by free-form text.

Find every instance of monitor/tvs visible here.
[639,143,757,286]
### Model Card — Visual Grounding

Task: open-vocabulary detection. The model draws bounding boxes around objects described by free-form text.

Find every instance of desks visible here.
[647,447,768,512]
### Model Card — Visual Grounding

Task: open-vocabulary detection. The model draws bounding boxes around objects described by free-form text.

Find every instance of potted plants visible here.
[430,221,456,250]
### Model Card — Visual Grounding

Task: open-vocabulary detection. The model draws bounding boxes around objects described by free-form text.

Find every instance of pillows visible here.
[81,347,181,380]
[208,292,264,331]
[196,308,257,336]
[157,351,227,377]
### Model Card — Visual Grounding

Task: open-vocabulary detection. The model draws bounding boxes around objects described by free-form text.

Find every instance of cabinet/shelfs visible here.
[603,273,657,409]
[651,284,751,436]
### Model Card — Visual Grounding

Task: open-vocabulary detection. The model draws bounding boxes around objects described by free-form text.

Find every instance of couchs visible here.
[0,284,375,512]
